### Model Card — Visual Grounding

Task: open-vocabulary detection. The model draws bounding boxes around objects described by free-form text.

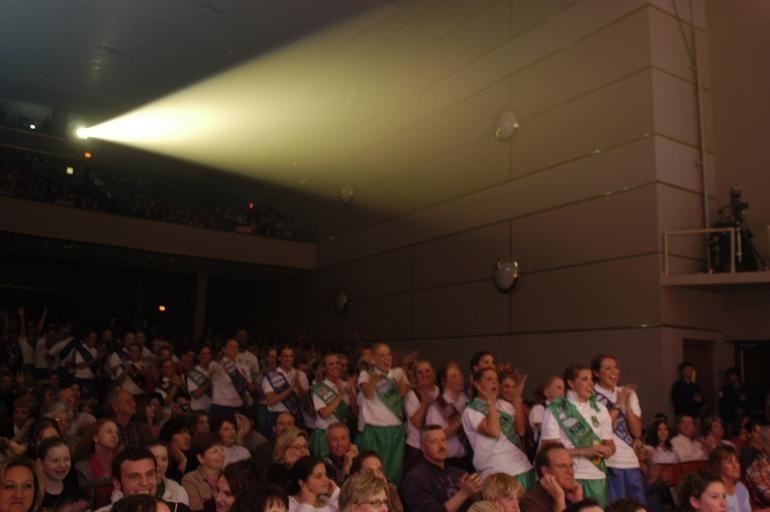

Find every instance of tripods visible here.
[712,222,770,274]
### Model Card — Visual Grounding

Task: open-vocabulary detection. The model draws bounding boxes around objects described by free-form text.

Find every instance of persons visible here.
[631,363,770,512]
[0,147,269,236]
[2,301,260,512]
[252,340,647,512]
[710,206,738,273]
